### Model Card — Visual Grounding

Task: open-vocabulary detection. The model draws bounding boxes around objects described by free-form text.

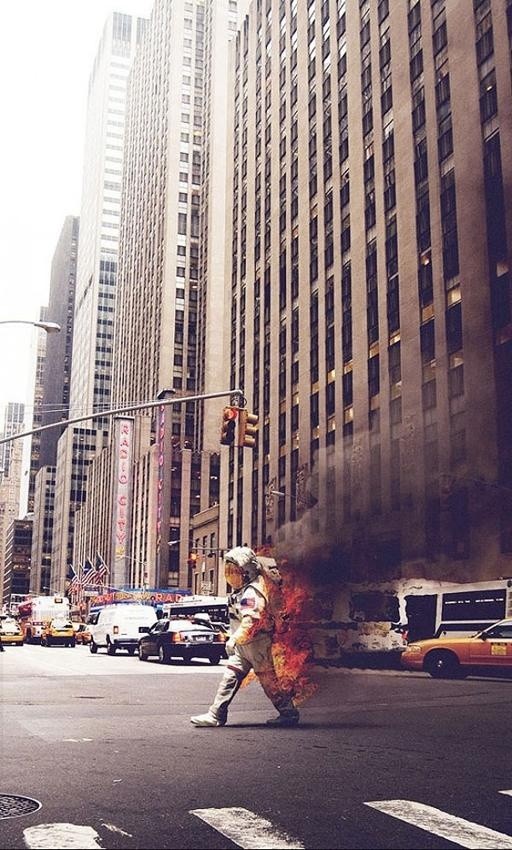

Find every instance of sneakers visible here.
[192,712,227,728]
[267,713,298,728]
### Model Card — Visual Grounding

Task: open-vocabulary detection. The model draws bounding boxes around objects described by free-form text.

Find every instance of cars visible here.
[0,614,24,646]
[40,619,94,647]
[401,618,512,680]
[138,619,227,666]
[89,604,158,655]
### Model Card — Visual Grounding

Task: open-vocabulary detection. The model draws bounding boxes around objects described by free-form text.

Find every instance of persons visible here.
[191,543,300,731]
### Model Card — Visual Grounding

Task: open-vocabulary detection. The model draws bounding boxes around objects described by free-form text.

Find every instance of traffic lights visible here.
[219,406,258,448]
[189,552,197,569]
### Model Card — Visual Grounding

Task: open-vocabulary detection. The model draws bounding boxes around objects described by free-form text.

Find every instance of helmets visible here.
[224,545,260,590]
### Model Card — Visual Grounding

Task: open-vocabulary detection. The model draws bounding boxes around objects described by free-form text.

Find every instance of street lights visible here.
[168,539,207,594]
[115,554,146,605]
[271,490,310,504]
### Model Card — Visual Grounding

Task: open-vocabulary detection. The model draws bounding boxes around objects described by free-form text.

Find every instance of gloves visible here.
[224,636,237,655]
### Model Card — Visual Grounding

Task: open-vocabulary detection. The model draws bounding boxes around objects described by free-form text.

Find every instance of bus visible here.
[19,596,70,645]
[293,584,407,666]
[161,595,232,638]
[405,578,512,647]
